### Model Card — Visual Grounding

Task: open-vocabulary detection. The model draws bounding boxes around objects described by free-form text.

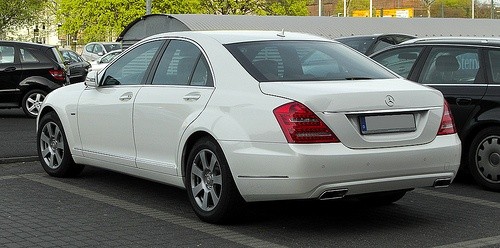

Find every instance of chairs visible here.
[431,55,462,85]
[138,51,279,88]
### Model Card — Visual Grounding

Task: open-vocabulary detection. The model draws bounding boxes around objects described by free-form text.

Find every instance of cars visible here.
[365,37,500,191]
[80,41,121,62]
[85,49,120,73]
[299,33,418,79]
[34,28,465,223]
[0,40,70,120]
[57,48,93,86]
